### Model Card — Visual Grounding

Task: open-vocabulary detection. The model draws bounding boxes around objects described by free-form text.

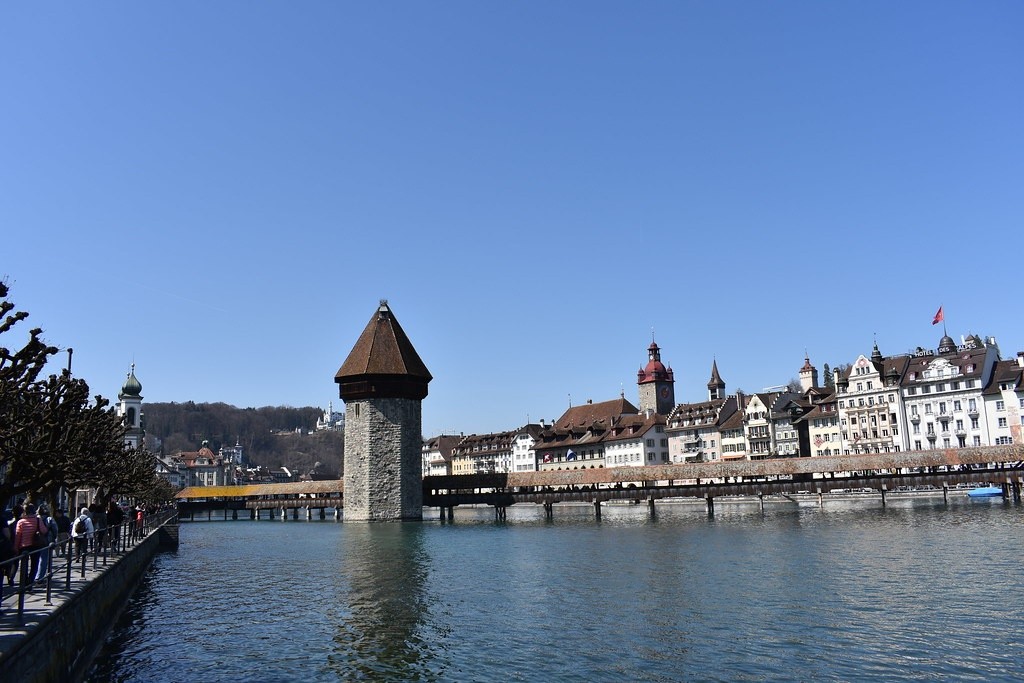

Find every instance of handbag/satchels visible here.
[32,519,49,550]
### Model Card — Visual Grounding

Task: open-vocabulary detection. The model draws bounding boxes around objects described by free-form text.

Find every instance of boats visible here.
[964,487,1004,497]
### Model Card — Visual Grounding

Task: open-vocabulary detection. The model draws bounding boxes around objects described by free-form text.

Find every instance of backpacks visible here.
[76,517,89,534]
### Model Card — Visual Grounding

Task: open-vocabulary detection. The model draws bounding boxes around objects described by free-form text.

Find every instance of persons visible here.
[1,498,176,589]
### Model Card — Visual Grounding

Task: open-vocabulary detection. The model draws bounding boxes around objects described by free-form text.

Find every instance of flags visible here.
[932,307,944,325]
[566,449,577,461]
[543,452,552,463]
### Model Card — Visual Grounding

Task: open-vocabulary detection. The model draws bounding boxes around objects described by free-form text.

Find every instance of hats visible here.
[39,505,51,514]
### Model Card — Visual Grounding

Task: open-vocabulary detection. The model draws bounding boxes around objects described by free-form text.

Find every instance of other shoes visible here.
[36,582,46,587]
[76,559,79,563]
[8,577,14,586]
[26,587,33,593]
